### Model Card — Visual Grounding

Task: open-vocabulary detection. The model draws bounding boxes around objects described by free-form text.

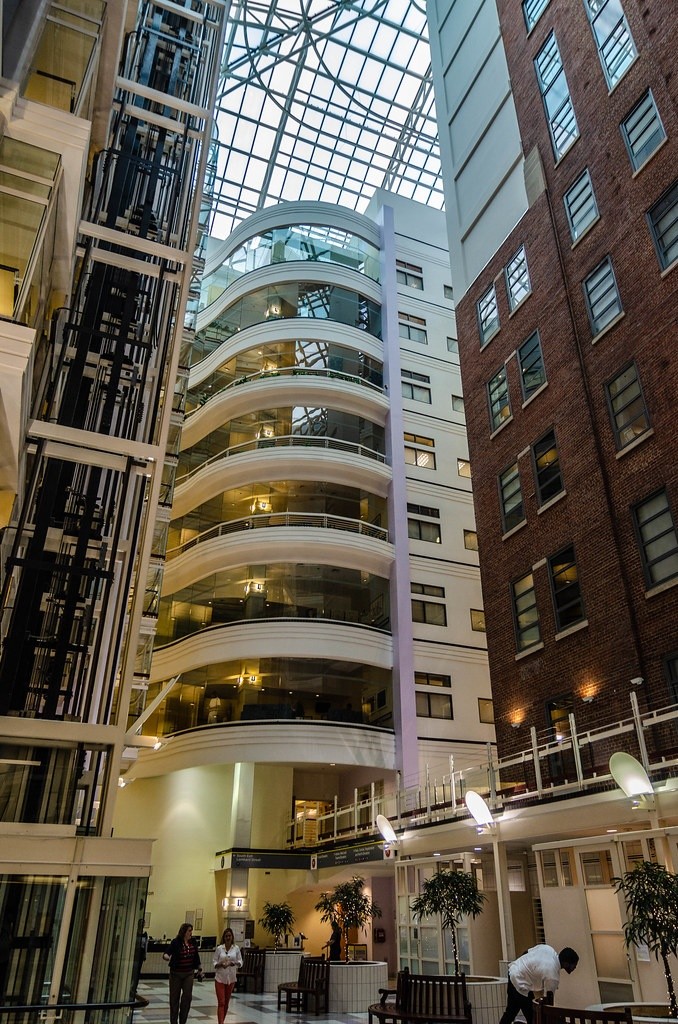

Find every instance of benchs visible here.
[368,966,473,1024]
[278,954,330,1017]
[532,991,633,1024]
[232,945,266,996]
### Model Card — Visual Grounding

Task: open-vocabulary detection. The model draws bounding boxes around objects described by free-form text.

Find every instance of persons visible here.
[132,919,149,993]
[207,690,221,723]
[213,928,244,1024]
[163,923,203,1024]
[202,392,207,400]
[499,944,580,1024]
[322,921,342,961]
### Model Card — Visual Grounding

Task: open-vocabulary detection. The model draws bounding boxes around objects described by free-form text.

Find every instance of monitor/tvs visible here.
[201,937,216,950]
[195,936,200,946]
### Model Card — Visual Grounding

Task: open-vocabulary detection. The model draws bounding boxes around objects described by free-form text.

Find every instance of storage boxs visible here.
[346,943,367,961]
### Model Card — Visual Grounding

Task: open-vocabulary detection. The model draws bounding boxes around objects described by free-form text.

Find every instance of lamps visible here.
[630,677,644,685]
[511,722,521,729]
[609,752,656,811]
[376,815,400,850]
[555,722,564,741]
[581,695,594,703]
[465,790,496,836]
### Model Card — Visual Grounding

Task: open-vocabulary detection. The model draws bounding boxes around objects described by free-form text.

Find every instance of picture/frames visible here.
[196,909,203,919]
[186,911,195,927]
[195,919,202,930]
[144,912,151,928]
